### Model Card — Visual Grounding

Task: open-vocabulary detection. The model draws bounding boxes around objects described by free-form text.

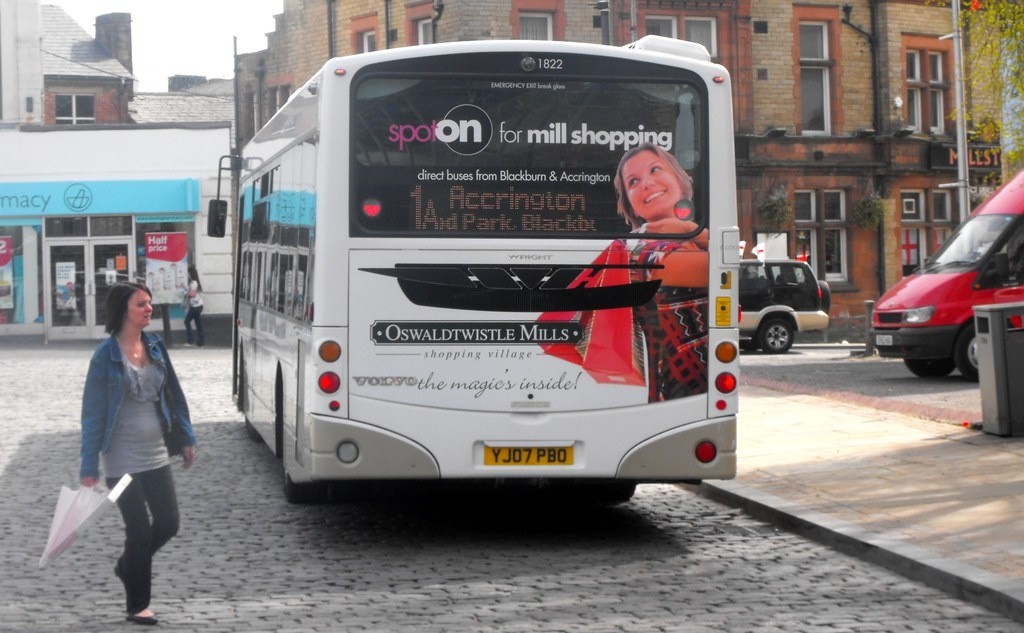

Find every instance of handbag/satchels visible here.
[537,238,645,386]
[38,481,113,568]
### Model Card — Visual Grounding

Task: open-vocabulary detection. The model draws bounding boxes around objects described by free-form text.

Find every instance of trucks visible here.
[869,170,1024,381]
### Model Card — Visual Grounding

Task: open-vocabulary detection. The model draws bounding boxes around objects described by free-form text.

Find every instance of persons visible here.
[183,266,206,349]
[612,141,708,403]
[79,281,195,623]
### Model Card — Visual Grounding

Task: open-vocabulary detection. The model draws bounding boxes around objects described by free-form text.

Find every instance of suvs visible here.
[738,260,831,355]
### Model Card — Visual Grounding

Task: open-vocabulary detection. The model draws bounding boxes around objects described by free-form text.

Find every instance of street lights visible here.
[939,33,971,254]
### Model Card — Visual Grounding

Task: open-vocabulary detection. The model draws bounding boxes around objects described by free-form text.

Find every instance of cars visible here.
[74,271,146,310]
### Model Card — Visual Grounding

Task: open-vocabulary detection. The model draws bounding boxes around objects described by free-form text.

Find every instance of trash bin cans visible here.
[971,300,1024,438]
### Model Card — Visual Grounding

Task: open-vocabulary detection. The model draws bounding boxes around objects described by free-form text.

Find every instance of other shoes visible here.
[183,343,194,347]
[127,608,159,625]
[114,560,125,582]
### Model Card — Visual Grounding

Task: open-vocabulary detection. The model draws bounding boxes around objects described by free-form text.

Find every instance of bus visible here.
[206,35,739,505]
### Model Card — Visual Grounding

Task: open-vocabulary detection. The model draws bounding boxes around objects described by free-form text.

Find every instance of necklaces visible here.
[132,352,139,359]
